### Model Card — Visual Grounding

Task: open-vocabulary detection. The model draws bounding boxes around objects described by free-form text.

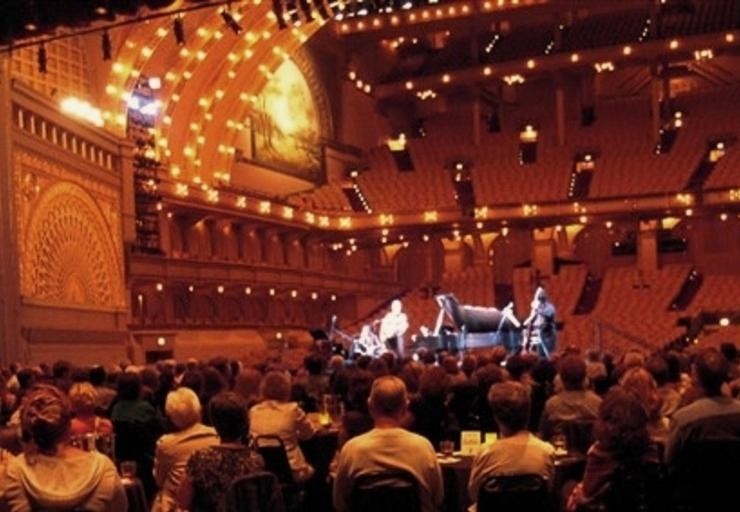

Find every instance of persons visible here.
[0,323,739,510]
[530,286,557,355]
[378,297,410,357]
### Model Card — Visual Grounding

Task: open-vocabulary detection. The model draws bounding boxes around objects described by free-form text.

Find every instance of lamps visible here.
[172,15,187,46]
[99,26,111,60]
[220,3,245,35]
[271,0,331,30]
[37,42,49,75]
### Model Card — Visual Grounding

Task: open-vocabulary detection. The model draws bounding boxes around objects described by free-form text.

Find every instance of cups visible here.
[121,460,138,482]
[440,440,455,458]
[552,434,567,453]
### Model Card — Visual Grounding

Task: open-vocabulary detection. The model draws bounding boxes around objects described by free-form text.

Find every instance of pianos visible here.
[411,294,519,352]
